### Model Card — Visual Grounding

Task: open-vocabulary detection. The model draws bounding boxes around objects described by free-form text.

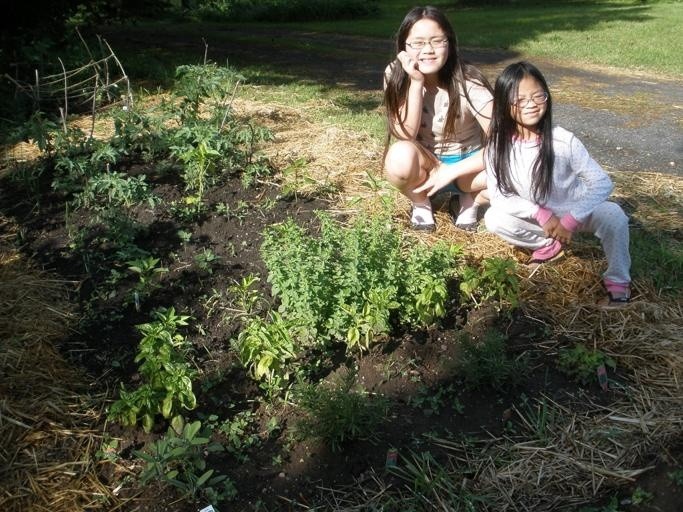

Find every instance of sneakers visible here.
[409,220,436,233]
[449,194,479,232]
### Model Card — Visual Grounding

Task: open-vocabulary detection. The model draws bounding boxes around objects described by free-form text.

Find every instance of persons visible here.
[484,61,632,307]
[380,5,494,235]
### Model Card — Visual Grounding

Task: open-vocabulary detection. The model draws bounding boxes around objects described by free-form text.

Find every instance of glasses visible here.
[405,38,448,48]
[511,92,548,108]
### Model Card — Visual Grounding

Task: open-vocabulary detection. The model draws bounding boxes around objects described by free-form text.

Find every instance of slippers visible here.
[525,250,565,268]
[596,291,629,307]
